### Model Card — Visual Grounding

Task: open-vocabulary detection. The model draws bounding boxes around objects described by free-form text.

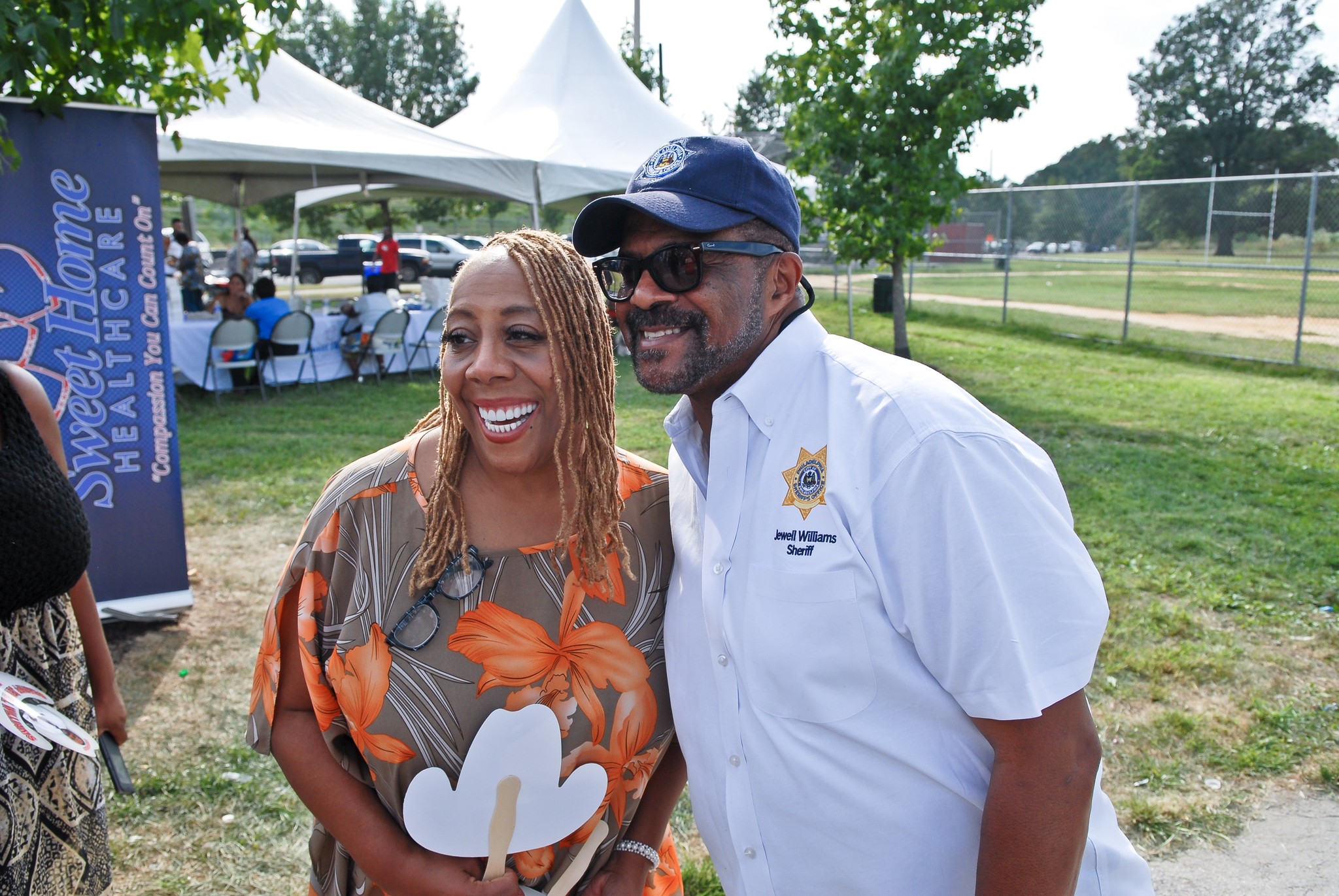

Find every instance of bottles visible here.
[305,296,312,316]
[214,300,224,321]
[323,296,331,315]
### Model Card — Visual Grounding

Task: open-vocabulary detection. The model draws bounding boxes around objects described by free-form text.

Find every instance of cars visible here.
[255,239,333,272]
[1025,240,1072,254]
[160,227,214,268]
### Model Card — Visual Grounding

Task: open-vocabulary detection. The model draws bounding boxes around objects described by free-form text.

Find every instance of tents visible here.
[290,0,711,310]
[151,43,543,403]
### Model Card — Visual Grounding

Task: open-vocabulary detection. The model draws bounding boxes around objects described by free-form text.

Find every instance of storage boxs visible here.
[362,261,383,279]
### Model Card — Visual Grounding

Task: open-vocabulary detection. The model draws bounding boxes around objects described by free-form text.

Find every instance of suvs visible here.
[392,233,493,279]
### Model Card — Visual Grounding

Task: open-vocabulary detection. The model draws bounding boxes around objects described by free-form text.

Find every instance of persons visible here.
[165,219,185,275]
[209,272,255,323]
[244,228,686,896]
[341,276,387,382]
[372,227,401,292]
[569,132,1152,896]
[229,277,293,390]
[0,359,130,896]
[168,233,208,312]
[225,224,257,286]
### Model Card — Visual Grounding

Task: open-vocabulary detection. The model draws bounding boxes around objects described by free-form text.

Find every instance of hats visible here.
[571,136,799,258]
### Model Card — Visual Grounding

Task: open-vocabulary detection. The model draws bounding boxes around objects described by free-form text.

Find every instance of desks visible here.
[172,310,446,394]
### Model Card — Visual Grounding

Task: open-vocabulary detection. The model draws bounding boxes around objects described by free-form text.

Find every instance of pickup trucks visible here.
[270,233,435,286]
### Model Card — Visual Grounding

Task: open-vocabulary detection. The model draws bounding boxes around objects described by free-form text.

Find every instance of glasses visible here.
[386,545,495,651]
[592,241,781,303]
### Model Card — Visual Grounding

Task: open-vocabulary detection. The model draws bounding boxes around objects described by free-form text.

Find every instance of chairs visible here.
[201,316,269,408]
[406,306,451,381]
[341,304,415,385]
[261,311,322,395]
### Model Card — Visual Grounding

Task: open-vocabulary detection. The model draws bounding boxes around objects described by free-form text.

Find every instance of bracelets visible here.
[615,840,660,872]
